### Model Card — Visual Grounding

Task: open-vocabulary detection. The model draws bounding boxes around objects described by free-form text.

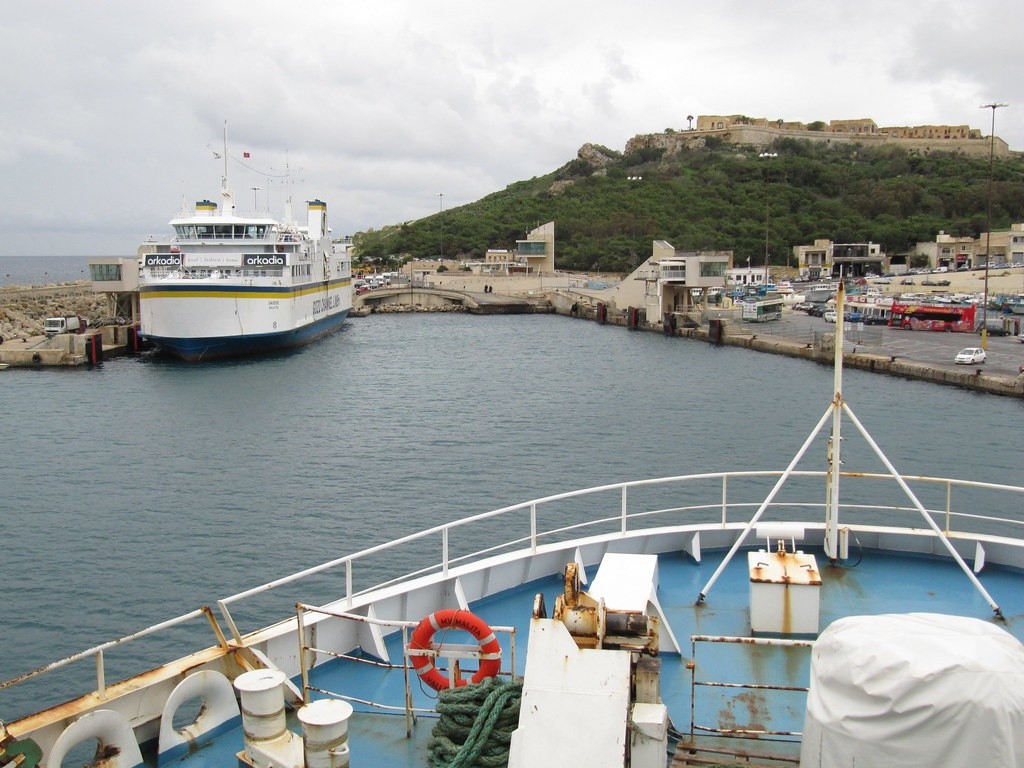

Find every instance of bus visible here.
[741,295,786,324]
[888,299,978,333]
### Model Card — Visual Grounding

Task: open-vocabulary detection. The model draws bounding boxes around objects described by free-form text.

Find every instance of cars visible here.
[854,254,1023,313]
[790,293,891,330]
[350,262,635,294]
[1017,331,1024,344]
[979,325,1012,338]
[954,346,987,365]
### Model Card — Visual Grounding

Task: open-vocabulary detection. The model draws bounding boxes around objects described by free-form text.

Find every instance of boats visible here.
[1007,292,1024,315]
[684,273,952,293]
[132,113,356,362]
[0,273,1023,766]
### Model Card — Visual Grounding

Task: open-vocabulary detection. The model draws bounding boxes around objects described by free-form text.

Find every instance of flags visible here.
[244,153,250,158]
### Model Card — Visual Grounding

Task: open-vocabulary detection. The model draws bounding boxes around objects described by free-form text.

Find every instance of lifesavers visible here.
[409,609,501,692]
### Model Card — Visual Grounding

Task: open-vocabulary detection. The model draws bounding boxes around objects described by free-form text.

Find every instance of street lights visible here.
[626,175,644,275]
[978,99,1010,341]
[436,191,446,269]
[758,148,779,293]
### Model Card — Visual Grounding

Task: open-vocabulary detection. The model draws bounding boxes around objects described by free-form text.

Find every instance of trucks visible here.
[43,312,88,337]
